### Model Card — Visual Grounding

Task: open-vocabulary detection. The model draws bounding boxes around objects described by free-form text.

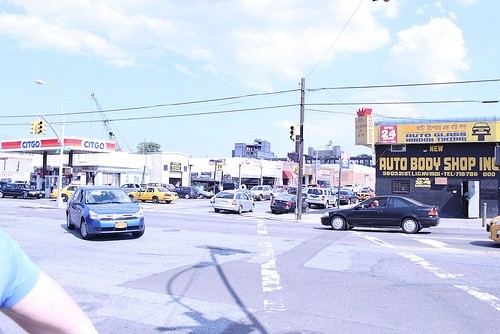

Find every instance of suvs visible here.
[303,187,338,209]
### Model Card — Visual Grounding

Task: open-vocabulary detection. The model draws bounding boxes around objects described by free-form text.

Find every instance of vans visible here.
[0,178,12,185]
[249,185,273,201]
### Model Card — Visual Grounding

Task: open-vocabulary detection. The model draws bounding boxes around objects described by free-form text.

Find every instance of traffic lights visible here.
[36,120,37,135]
[289,124,296,141]
[43,121,48,135]
[36,120,43,134]
[29,121,35,134]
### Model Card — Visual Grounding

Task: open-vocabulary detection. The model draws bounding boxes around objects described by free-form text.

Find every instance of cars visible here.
[127,186,179,204]
[356,189,375,202]
[66,185,146,240]
[334,189,359,206]
[0,183,41,199]
[320,195,439,234]
[270,193,310,214]
[193,185,215,200]
[174,185,200,200]
[209,189,255,214]
[49,184,83,202]
[272,185,298,195]
[120,182,145,194]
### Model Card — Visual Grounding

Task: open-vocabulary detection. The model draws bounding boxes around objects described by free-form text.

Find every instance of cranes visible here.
[89,93,121,152]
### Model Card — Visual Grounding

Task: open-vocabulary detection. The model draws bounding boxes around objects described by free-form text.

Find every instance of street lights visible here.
[36,79,65,207]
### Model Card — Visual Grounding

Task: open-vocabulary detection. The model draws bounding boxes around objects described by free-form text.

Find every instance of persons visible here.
[372,200,381,209]
[0,224,99,334]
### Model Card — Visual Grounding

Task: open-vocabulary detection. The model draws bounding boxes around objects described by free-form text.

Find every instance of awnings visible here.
[283,170,293,179]
